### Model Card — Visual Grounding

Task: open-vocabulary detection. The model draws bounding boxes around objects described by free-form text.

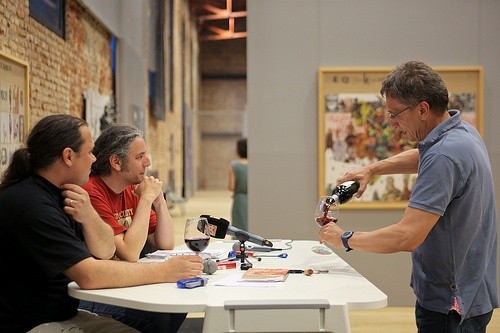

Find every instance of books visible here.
[242,268,289,282]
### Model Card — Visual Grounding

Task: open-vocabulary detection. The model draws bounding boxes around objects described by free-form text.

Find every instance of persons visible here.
[0,115,204,333]
[319,61,500,333]
[229,138,248,230]
[79,124,186,333]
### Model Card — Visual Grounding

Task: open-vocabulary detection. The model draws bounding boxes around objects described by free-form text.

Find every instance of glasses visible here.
[388,105,413,121]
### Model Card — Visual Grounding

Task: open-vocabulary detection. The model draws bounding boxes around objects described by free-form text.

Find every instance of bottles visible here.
[326,181,360,205]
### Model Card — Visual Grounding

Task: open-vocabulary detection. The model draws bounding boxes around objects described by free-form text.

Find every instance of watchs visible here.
[341,231,354,252]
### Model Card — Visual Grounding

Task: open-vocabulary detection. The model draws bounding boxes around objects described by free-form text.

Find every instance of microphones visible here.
[199,215,273,247]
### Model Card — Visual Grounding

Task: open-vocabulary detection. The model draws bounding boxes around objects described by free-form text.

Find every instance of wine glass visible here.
[183,218,211,280]
[310,196,340,256]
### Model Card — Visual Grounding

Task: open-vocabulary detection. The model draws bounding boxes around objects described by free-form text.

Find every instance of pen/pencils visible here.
[289,270,304,273]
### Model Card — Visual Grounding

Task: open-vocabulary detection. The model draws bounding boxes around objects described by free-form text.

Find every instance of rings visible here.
[70,200,73,205]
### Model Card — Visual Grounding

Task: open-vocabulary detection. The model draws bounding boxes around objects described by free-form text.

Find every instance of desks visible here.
[67,239,389,333]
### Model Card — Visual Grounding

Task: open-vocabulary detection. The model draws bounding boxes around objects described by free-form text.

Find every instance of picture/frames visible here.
[314,67,485,213]
[0,52,30,179]
